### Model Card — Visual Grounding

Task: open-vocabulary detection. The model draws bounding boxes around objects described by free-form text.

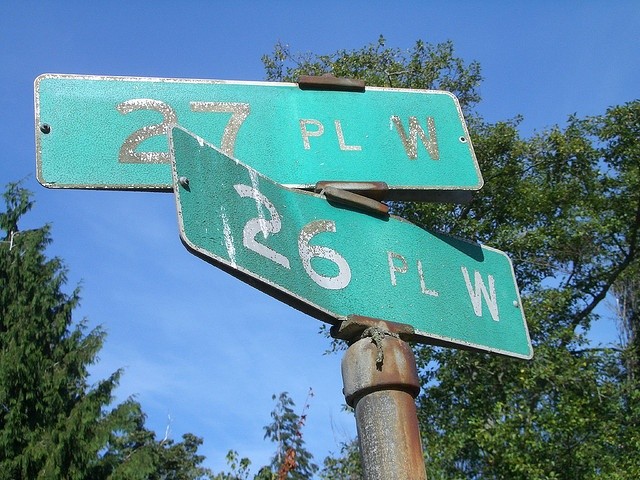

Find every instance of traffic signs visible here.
[167,121,534,362]
[34,73,485,205]
